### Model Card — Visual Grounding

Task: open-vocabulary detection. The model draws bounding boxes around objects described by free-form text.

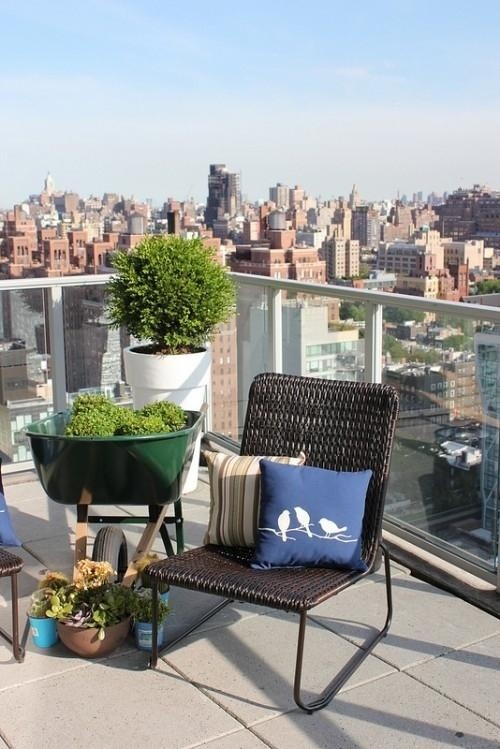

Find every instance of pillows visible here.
[250,459,373,569]
[0,487,22,545]
[203,450,306,555]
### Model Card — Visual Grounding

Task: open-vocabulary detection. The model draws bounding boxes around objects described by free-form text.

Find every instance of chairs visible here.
[0,547,25,663]
[142,373,399,712]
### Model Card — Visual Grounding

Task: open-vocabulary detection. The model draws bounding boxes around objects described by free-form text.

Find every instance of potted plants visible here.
[27,587,59,648]
[106,233,241,494]
[25,390,207,505]
[45,558,135,656]
[136,573,171,651]
[43,571,66,587]
